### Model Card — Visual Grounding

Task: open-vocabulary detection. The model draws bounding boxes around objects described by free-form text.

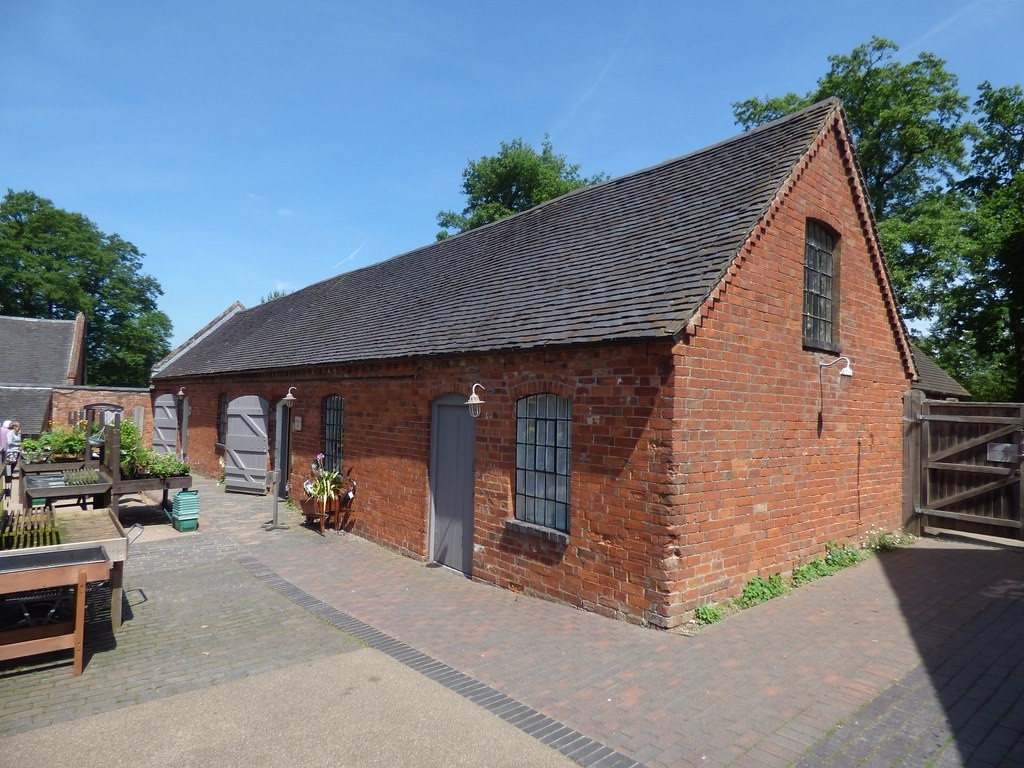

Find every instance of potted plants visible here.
[118,419,192,478]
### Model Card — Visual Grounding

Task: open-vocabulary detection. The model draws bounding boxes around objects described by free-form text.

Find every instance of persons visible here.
[0,420,25,480]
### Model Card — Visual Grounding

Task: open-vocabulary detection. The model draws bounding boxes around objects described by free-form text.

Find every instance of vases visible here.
[301,496,338,515]
[21,452,80,463]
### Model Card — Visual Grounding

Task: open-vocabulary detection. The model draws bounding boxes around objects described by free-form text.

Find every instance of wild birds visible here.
[339,465,357,532]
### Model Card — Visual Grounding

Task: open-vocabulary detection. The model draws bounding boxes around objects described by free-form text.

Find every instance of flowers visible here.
[303,454,344,515]
[21,418,88,454]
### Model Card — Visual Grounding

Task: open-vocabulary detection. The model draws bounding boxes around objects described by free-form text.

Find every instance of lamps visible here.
[177,387,186,400]
[282,387,298,408]
[818,356,852,377]
[463,383,485,417]
